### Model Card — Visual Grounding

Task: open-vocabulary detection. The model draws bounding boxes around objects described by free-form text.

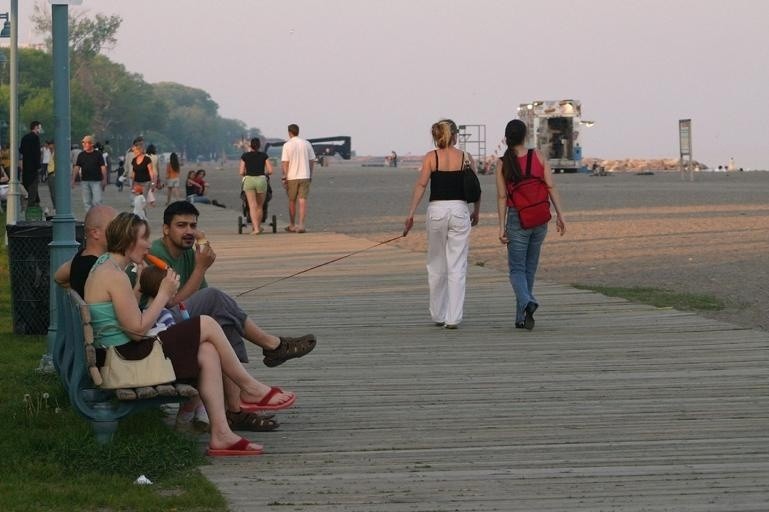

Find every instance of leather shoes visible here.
[514,321,525,329]
[523,299,538,330]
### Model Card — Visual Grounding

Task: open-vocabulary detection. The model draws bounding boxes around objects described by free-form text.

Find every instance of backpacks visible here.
[499,147,552,230]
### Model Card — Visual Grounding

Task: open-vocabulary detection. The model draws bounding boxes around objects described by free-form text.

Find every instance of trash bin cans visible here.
[6,223,87,335]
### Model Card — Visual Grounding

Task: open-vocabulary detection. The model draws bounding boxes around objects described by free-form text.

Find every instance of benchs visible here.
[50,284,202,443]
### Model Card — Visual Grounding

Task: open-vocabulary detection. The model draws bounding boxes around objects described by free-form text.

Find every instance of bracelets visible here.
[282,177,286,180]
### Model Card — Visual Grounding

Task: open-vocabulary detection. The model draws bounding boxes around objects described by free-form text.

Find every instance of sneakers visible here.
[251,227,265,236]
[194,406,211,433]
[171,410,195,433]
[284,226,307,234]
[435,322,459,330]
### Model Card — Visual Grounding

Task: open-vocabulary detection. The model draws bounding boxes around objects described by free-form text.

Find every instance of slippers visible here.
[204,433,265,456]
[238,385,297,413]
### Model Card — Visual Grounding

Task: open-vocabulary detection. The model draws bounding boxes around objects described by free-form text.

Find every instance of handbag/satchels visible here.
[460,150,482,205]
[96,338,179,391]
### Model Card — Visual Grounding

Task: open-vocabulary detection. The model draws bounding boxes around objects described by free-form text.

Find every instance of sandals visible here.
[261,333,319,367]
[224,409,284,431]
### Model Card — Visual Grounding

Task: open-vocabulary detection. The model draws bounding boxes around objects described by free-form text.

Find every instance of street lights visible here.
[0,10,14,41]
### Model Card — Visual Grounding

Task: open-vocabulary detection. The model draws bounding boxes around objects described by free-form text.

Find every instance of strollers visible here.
[235,174,282,237]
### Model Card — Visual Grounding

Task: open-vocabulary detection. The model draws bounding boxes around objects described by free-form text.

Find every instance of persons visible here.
[384,151,398,167]
[194,170,206,195]
[19,120,42,205]
[165,153,181,204]
[0,137,160,207]
[131,185,146,219]
[142,201,317,433]
[404,117,481,329]
[131,142,156,216]
[186,170,211,204]
[53,205,117,298]
[72,135,108,212]
[477,160,496,175]
[85,212,292,451]
[241,138,273,234]
[141,263,210,429]
[592,161,601,176]
[496,119,565,330]
[44,139,57,214]
[281,124,316,233]
